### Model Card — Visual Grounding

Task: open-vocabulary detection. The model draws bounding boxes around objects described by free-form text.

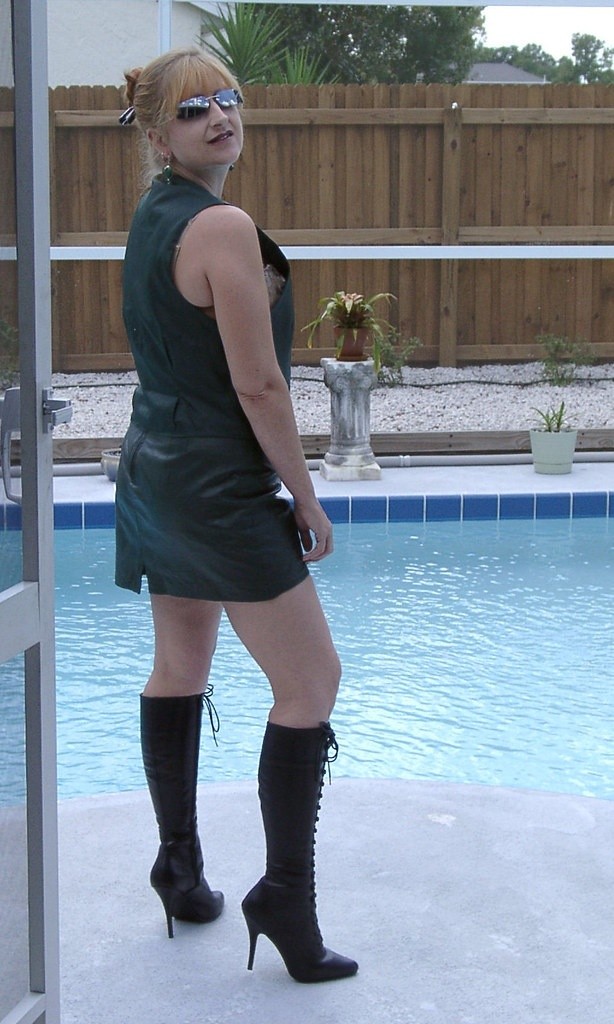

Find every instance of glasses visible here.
[175,88,242,119]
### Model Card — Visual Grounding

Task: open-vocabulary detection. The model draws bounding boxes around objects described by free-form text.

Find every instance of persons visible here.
[112,45,362,986]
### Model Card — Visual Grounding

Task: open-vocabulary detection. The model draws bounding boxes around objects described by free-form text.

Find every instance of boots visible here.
[240,720,359,983]
[140,682,224,938]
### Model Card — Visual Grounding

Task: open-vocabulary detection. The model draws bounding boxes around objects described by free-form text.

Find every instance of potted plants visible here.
[300,290,398,372]
[526,401,579,474]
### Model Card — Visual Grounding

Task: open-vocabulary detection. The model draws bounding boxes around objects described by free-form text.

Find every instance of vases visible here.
[101,449,123,482]
